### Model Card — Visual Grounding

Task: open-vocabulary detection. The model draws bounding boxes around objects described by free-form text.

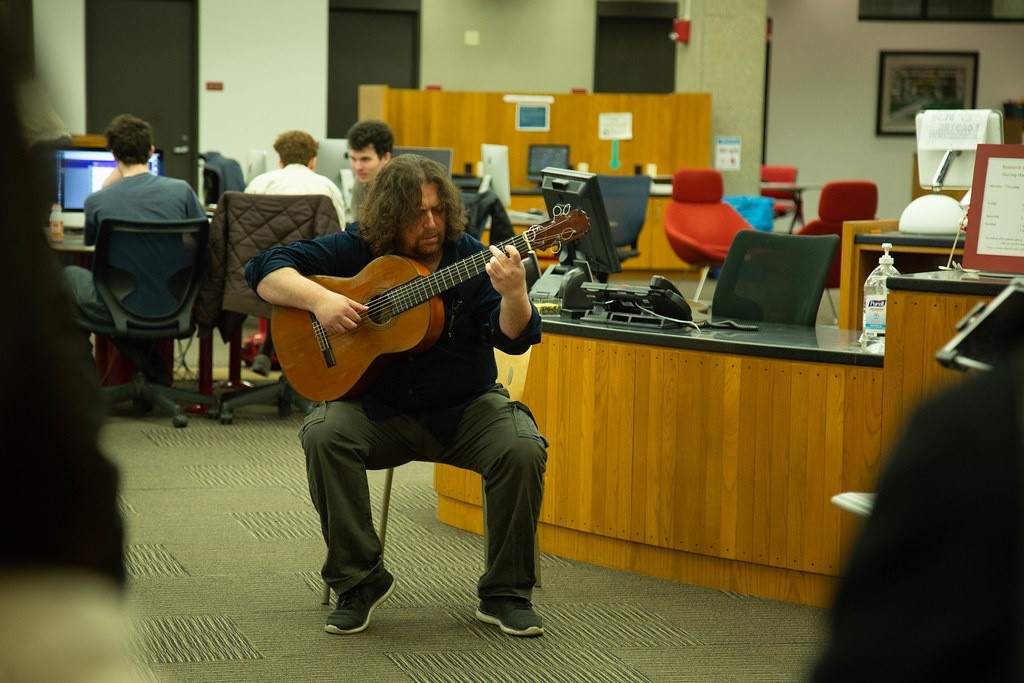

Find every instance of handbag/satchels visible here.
[93,328,175,389]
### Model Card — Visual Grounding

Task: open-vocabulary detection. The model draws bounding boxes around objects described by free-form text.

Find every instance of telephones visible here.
[580,275,693,332]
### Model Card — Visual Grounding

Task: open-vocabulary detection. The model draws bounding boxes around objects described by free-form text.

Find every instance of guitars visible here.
[272,202,590,403]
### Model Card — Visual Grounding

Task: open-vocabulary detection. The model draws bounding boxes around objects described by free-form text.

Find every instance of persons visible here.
[347,119,394,221]
[245,154,551,635]
[65,114,208,323]
[0,69,131,683]
[244,130,345,232]
[796,365,1024,683]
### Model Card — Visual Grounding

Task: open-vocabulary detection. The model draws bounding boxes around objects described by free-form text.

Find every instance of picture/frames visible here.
[875,50,980,135]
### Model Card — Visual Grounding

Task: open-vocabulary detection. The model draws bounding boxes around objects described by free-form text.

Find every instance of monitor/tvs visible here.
[479,144,624,275]
[312,138,357,212]
[915,109,1004,190]
[52,145,164,230]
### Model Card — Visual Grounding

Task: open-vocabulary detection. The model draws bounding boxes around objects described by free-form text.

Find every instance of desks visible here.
[478,207,544,263]
[839,214,972,332]
[764,180,823,229]
[50,224,248,416]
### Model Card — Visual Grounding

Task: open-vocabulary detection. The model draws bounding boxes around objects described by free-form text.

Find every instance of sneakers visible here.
[476,594,544,637]
[323,564,396,634]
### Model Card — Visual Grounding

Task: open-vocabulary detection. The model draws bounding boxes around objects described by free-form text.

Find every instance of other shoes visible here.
[252,354,273,377]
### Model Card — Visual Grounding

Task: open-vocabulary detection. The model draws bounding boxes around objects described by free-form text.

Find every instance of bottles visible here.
[49,204,63,242]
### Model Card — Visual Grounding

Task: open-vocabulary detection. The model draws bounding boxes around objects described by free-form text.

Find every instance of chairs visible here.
[797,180,879,323]
[319,442,544,602]
[591,172,653,263]
[662,167,756,304]
[216,189,344,426]
[76,215,219,427]
[760,165,808,235]
[711,226,839,333]
